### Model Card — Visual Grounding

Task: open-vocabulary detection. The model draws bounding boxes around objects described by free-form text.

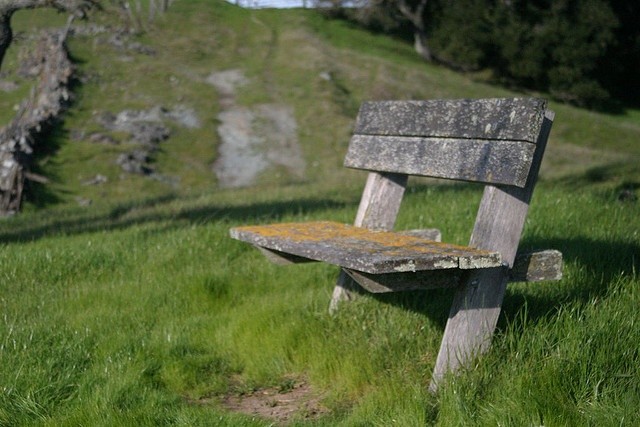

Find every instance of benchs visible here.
[229,96,563,397]
